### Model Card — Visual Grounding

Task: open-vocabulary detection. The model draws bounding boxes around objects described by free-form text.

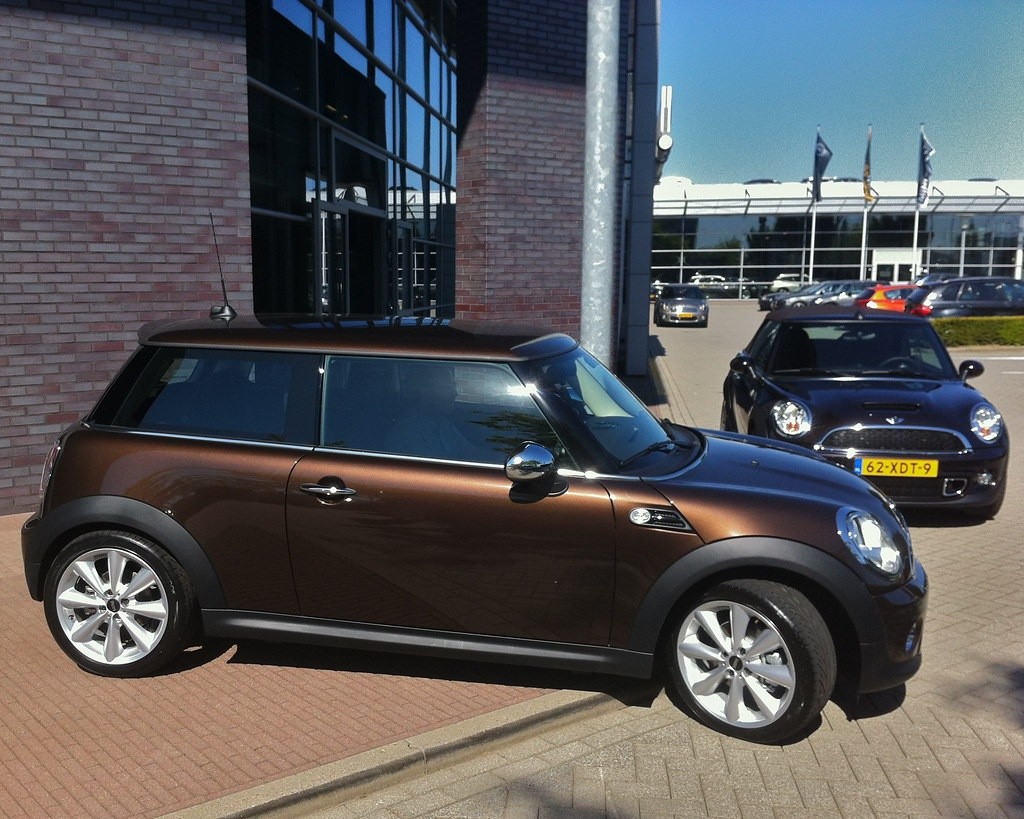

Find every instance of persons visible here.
[387,366,484,459]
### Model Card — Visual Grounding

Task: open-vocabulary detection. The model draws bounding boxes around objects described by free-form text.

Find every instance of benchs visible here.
[811,339,835,354]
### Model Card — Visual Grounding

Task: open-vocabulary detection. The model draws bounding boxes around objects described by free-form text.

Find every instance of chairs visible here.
[774,328,812,369]
[834,332,868,367]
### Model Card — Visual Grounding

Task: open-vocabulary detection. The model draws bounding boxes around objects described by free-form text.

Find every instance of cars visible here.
[723,307,1009,518]
[758,274,1024,318]
[654,285,709,327]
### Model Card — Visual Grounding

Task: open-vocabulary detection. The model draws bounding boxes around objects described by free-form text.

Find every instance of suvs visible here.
[688,274,730,288]
[21,213,928,744]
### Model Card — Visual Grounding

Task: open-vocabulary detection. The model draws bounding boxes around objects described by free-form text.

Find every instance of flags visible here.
[916,130,938,206]
[862,130,877,206]
[812,133,834,201]
